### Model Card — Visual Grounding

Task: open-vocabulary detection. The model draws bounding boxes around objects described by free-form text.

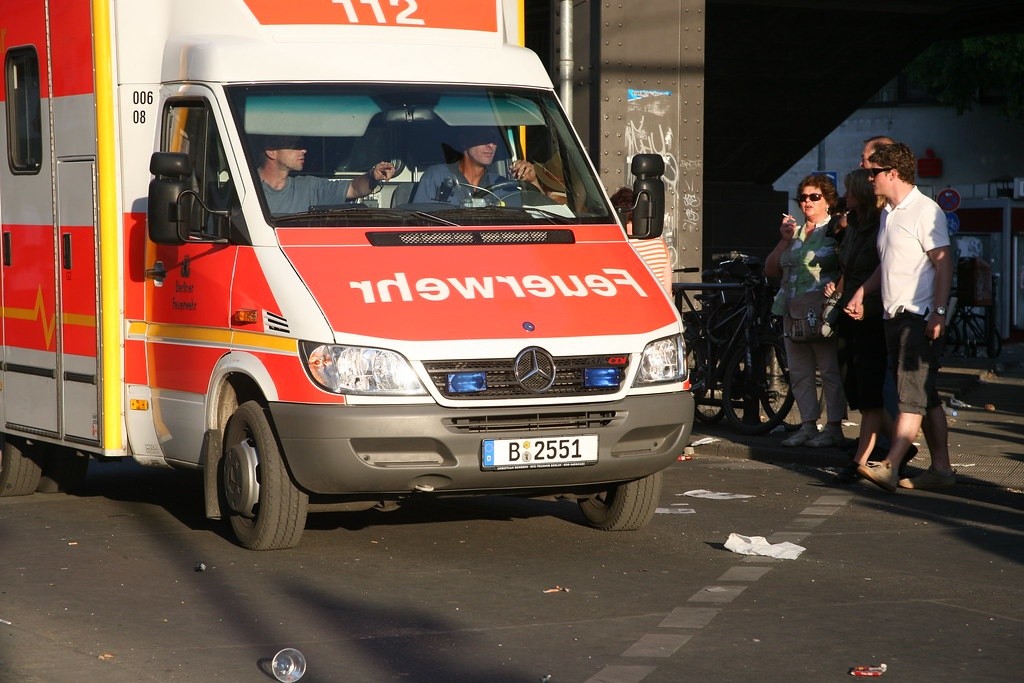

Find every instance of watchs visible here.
[931,306,947,315]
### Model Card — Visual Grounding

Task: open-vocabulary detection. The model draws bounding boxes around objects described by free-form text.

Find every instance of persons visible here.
[764,136,957,494]
[610,187,673,301]
[258,134,396,220]
[412,127,546,205]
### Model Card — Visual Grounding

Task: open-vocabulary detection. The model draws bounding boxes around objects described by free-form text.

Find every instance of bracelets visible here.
[368,165,378,182]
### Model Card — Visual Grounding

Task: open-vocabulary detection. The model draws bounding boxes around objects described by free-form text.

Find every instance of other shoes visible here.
[868,446,889,459]
[832,461,864,484]
[899,444,918,469]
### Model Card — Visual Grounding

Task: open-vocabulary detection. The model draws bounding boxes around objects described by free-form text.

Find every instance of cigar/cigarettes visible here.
[512,161,516,173]
[783,213,796,222]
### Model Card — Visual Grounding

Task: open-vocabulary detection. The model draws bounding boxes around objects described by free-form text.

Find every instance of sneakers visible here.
[899,464,955,489]
[857,460,898,492]
[782,423,818,446]
[804,424,845,448]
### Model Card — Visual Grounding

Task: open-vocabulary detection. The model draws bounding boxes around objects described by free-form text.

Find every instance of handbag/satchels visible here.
[783,291,838,344]
[830,296,882,338]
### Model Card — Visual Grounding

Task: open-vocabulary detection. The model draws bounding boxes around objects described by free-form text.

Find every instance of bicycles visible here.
[669,249,827,437]
[931,246,1004,359]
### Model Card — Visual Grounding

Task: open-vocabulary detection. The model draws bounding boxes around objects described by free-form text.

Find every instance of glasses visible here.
[869,166,898,178]
[796,193,824,202]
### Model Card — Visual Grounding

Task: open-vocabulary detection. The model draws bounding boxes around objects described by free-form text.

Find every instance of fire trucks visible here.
[0,0,697,551]
